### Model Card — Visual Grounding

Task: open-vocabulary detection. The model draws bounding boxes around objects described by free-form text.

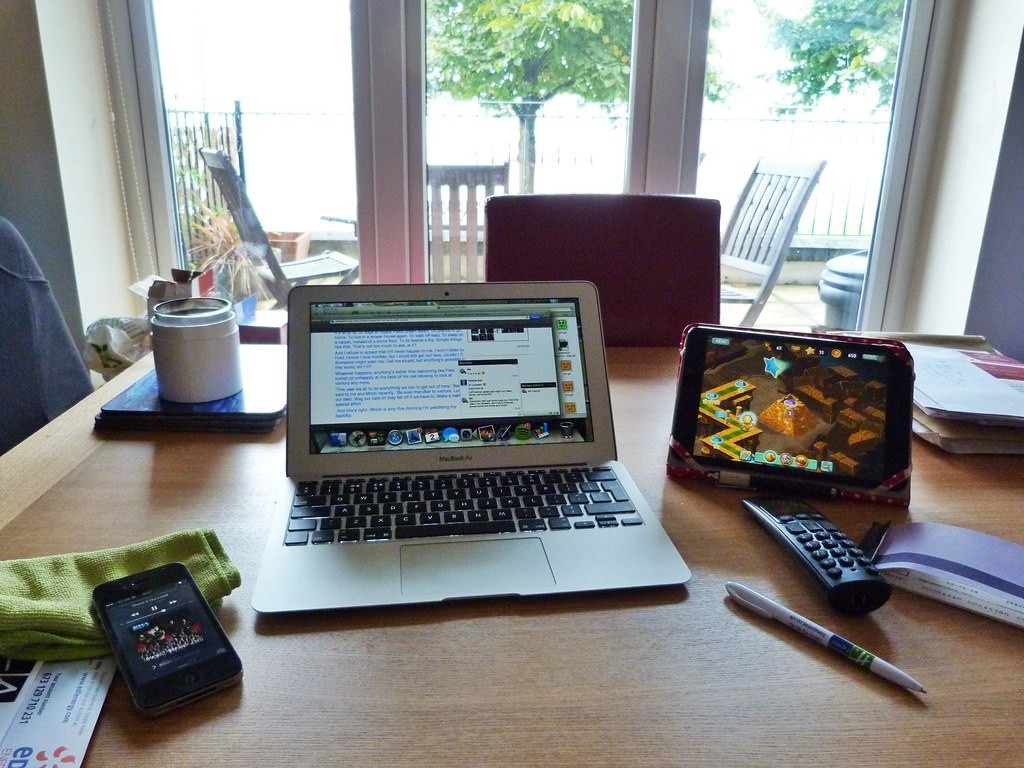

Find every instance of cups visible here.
[149,298,243,404]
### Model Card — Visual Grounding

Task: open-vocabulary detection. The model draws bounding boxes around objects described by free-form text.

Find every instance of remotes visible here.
[743,497,893,616]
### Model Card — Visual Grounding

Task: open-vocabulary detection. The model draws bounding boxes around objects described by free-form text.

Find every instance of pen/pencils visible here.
[725,580,928,699]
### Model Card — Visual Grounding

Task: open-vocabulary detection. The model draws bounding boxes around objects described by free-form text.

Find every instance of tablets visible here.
[667,322,914,506]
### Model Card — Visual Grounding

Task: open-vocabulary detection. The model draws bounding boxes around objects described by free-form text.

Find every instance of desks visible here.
[321,215,483,286]
[0,345,1023,767]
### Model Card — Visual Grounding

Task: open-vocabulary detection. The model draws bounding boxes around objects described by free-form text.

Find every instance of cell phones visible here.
[92,563,244,718]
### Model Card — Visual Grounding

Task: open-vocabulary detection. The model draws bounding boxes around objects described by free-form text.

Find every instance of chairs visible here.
[720,157,828,328]
[427,162,510,283]
[484,194,721,345]
[0,215,94,459]
[200,148,359,310]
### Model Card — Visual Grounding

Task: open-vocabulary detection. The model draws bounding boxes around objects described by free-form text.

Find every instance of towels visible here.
[0,527,242,662]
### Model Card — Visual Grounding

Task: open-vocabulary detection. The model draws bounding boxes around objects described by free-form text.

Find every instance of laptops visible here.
[251,280,692,613]
